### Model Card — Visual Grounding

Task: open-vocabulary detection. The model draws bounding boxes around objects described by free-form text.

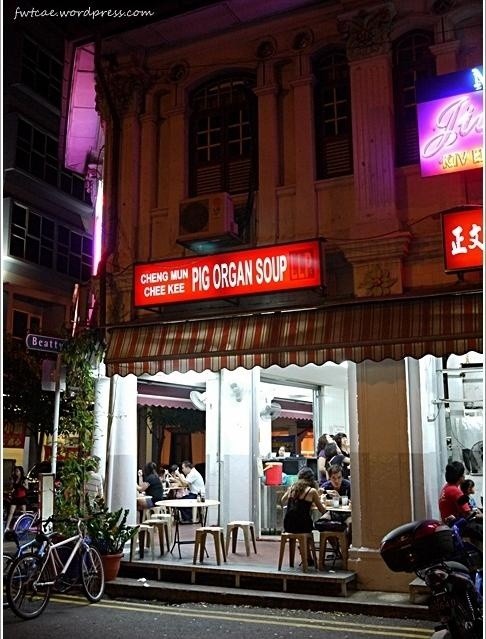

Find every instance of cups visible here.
[333,496,340,507]
[342,496,349,506]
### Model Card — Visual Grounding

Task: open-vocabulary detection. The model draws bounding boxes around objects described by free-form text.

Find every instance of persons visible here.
[276,432,351,565]
[138,458,206,524]
[78,455,105,518]
[3,465,29,533]
[438,459,482,526]
[463,479,478,511]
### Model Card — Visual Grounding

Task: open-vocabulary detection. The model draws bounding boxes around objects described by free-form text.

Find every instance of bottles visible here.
[197,495,200,502]
[201,487,206,502]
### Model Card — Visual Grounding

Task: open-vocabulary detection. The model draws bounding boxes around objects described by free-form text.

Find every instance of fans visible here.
[188,386,209,414]
[256,400,285,422]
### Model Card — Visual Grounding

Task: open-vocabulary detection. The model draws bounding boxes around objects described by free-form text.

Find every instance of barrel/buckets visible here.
[264,462,283,486]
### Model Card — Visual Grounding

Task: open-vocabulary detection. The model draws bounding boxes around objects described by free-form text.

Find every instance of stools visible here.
[122,469,354,573]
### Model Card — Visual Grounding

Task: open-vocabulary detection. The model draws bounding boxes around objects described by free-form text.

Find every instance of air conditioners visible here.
[170,187,237,241]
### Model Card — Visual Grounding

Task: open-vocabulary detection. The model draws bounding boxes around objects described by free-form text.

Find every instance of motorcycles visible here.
[379,506,484,639]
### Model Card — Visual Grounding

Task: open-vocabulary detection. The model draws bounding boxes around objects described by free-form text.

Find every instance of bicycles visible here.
[6,506,106,620]
[2,517,64,608]
[12,490,39,542]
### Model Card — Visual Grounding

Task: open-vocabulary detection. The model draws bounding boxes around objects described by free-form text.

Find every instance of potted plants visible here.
[80,498,137,583]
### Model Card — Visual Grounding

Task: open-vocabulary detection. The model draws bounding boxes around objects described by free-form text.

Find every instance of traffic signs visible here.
[26,333,68,355]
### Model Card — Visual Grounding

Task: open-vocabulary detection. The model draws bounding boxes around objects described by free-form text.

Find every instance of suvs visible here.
[12,461,65,507]
